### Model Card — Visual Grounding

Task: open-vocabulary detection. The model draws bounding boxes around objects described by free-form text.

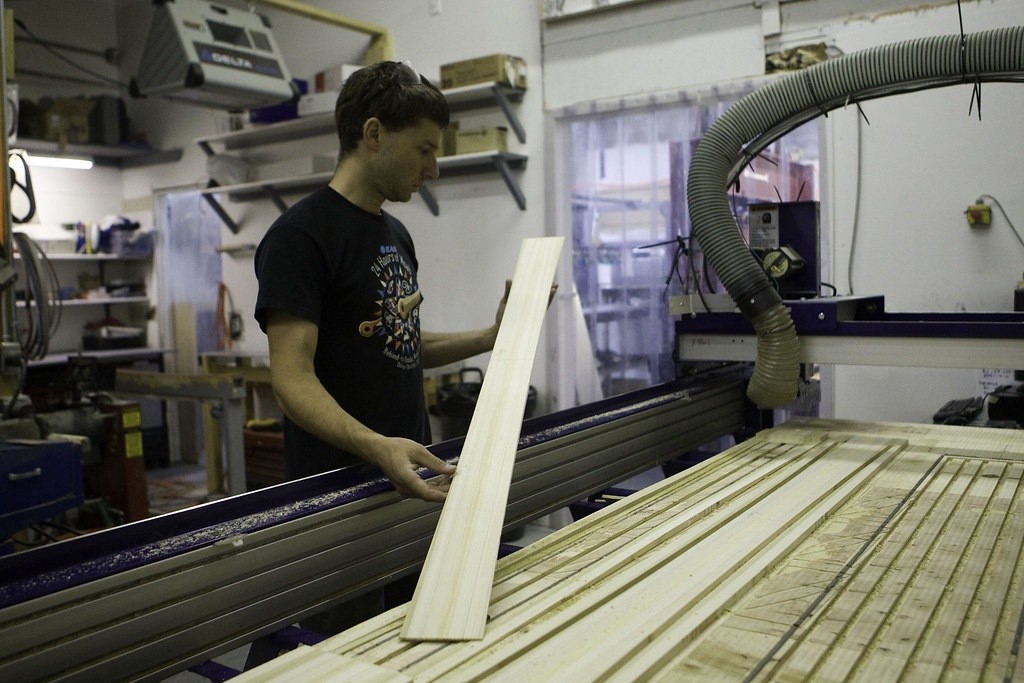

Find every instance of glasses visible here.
[358,59,422,110]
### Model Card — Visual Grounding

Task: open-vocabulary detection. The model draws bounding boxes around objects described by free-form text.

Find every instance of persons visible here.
[253,59,560,646]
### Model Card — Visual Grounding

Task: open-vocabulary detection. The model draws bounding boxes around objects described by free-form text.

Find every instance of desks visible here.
[26,348,176,467]
[199,350,442,496]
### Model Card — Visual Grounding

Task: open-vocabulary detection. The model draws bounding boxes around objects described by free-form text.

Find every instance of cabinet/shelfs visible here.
[194,81,528,235]
[13,253,159,306]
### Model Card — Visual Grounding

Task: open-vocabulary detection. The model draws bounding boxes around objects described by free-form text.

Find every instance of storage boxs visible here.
[443,120,508,157]
[441,53,527,90]
[109,231,151,255]
[26,97,96,144]
[248,154,334,182]
[228,76,339,130]
[293,64,364,91]
